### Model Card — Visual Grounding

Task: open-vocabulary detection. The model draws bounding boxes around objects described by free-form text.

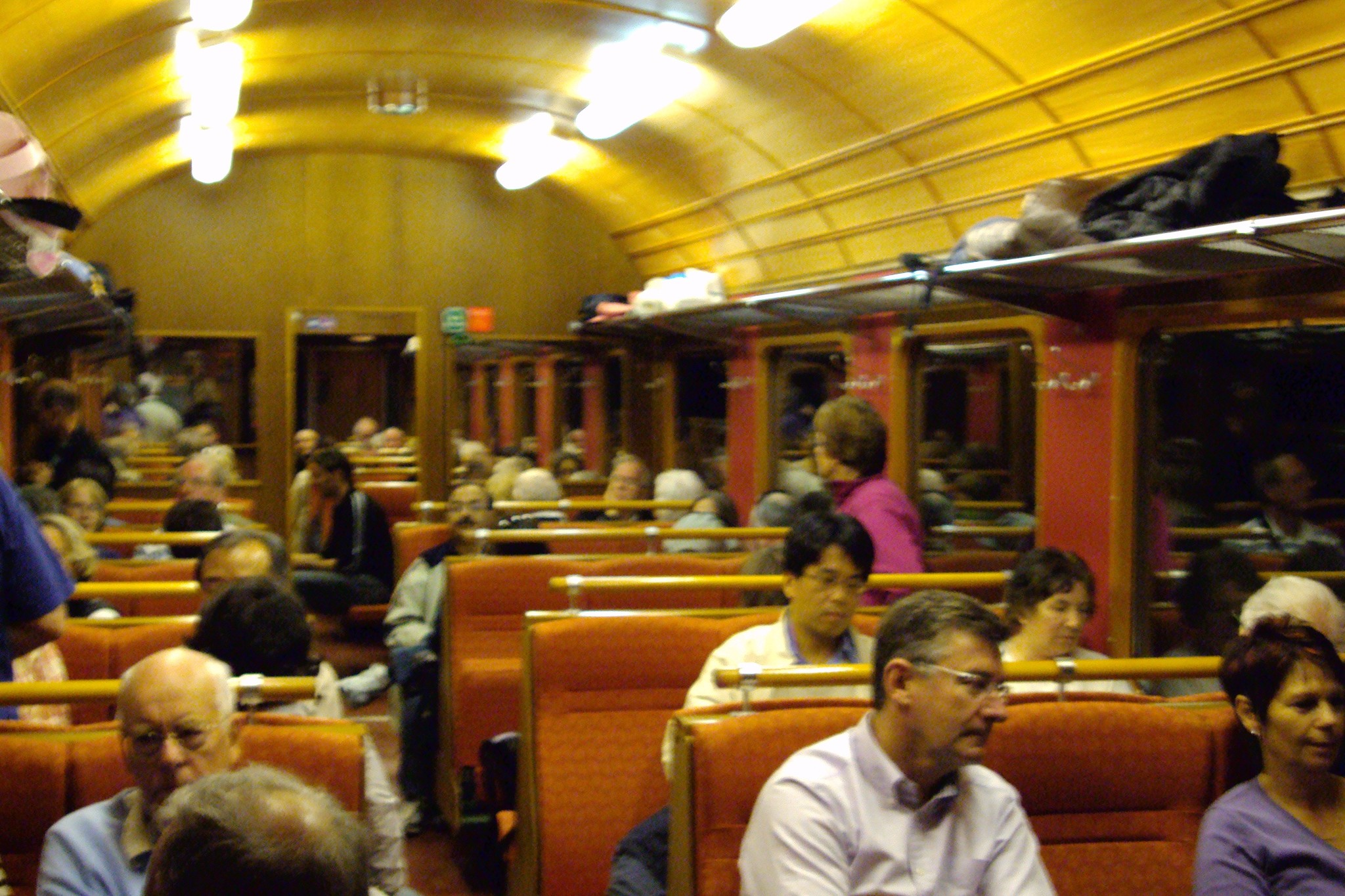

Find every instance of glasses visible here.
[913,660,1009,695]
[795,570,869,594]
[122,722,231,755]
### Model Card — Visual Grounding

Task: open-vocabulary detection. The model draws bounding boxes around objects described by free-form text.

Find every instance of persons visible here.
[0,366,840,721]
[35,642,248,896]
[135,766,389,896]
[659,510,888,789]
[925,450,1344,589]
[806,392,931,604]
[738,585,1063,895]
[987,548,1142,700]
[389,482,510,836]
[1195,613,1345,896]
[1236,576,1345,653]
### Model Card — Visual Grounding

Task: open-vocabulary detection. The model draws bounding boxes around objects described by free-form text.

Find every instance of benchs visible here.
[23,428,636,557]
[0,673,369,896]
[53,581,321,725]
[513,573,1029,896]
[672,656,1267,896]
[392,500,716,596]
[62,562,215,622]
[438,528,752,837]
